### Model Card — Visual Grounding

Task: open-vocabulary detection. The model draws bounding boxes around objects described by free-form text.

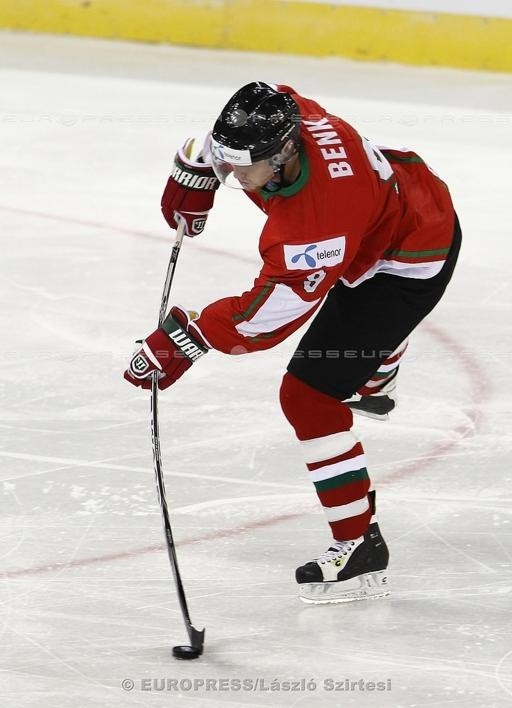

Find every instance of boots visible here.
[340,364,404,416]
[294,491,390,584]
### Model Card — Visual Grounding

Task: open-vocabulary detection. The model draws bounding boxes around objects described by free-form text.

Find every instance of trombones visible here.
[150,217,205,656]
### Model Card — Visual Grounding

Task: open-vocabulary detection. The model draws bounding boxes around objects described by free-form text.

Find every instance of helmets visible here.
[202,80,302,190]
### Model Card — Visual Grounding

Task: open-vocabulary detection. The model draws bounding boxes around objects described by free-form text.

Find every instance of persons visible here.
[122,80,462,587]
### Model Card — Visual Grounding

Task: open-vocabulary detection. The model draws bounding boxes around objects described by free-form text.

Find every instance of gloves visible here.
[123,304,213,391]
[160,135,226,239]
